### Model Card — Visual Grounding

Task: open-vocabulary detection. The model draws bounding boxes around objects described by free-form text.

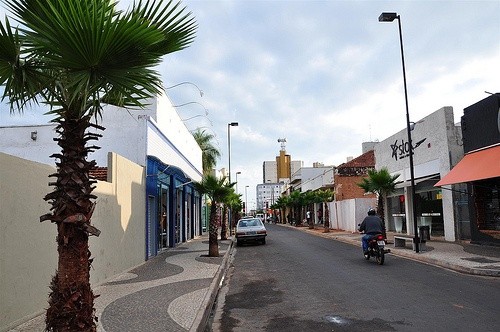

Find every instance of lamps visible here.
[162,82,204,97]
[173,101,215,137]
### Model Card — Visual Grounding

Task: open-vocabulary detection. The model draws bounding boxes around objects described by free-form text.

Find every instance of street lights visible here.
[227,122,238,185]
[245,186,249,216]
[236,172,241,194]
[379,12,419,253]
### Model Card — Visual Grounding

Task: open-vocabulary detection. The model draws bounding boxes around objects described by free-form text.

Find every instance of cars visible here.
[236,213,267,245]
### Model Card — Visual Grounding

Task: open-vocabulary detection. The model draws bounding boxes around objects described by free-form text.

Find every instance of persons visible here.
[317,208,322,224]
[307,210,311,226]
[287,213,290,223]
[358,208,385,258]
[267,214,270,223]
[276,214,280,222]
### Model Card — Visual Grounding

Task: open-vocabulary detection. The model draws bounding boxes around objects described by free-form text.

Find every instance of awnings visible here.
[434,145,500,186]
[364,174,439,195]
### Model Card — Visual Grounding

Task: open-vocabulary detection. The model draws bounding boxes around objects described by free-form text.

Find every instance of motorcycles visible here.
[357,224,390,265]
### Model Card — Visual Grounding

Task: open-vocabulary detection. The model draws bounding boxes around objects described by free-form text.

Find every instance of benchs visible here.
[393,236,421,251]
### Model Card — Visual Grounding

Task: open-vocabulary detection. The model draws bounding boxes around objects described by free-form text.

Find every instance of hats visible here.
[367,207,375,212]
